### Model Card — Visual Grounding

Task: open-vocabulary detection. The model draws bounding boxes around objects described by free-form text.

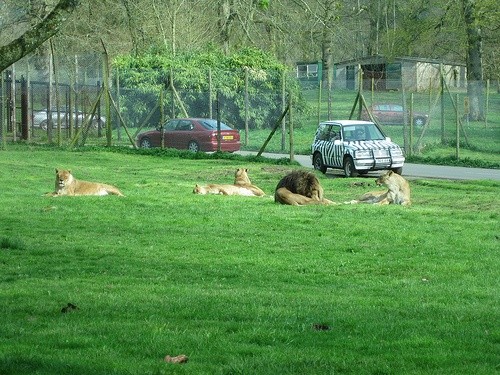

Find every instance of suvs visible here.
[310,120,405,177]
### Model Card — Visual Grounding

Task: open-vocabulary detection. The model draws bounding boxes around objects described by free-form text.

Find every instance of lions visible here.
[192,168,266,197]
[275,170,341,206]
[344,170,411,205]
[40,168,126,197]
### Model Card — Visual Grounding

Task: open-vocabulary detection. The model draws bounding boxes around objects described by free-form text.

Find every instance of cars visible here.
[29,104,106,130]
[358,103,429,127]
[136,118,240,153]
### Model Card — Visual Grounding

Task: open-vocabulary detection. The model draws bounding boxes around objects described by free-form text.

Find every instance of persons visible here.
[317,131,341,142]
[355,129,365,141]
